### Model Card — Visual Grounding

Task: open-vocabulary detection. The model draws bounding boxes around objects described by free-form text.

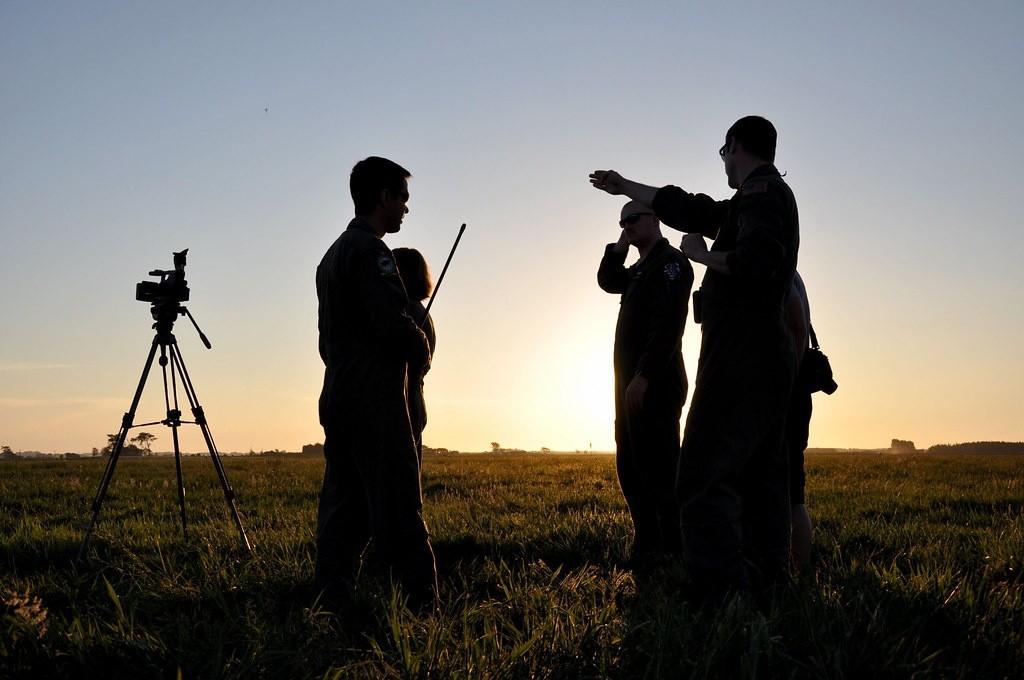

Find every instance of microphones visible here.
[149,269,174,276]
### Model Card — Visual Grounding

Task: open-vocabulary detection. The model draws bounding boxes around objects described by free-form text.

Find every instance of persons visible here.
[316,157,483,644]
[588,115,813,641]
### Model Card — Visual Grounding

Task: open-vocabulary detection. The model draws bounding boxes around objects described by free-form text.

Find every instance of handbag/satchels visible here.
[803,323,836,396]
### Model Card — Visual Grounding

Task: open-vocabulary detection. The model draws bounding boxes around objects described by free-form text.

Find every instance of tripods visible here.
[76,303,254,565]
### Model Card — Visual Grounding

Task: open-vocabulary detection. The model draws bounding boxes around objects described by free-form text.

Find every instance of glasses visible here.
[397,187,408,201]
[719,139,732,162]
[620,212,654,228]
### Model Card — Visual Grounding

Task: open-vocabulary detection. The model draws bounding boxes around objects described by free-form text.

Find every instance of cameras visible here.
[136,248,190,303]
